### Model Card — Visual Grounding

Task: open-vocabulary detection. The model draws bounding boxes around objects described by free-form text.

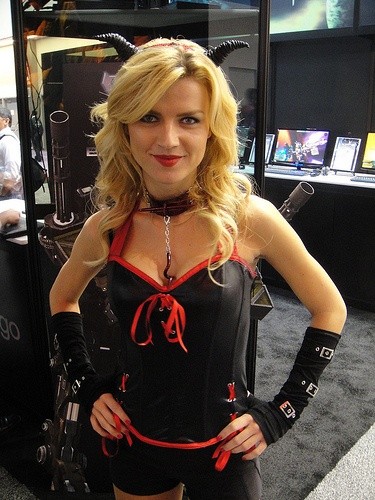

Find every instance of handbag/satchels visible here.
[37,361,99,500]
[0,134,45,192]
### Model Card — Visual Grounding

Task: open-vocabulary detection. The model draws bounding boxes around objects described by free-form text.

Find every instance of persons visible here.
[240,88,257,128]
[0,108,23,200]
[0,209,20,227]
[30,111,44,162]
[49,38,348,500]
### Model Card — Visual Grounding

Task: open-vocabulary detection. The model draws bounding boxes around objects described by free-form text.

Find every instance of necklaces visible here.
[142,185,195,280]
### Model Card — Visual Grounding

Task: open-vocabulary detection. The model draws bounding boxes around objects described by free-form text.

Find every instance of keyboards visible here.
[350,175,375,183]
[264,168,308,176]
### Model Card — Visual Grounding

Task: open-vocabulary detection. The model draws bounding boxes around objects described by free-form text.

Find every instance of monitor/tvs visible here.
[236,124,250,161]
[360,132,375,172]
[271,127,331,170]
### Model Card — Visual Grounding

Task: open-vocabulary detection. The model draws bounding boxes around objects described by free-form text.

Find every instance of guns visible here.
[33,108,318,500]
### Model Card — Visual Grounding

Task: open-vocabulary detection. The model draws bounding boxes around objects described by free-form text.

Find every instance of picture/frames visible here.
[330,137,360,172]
[251,133,276,164]
